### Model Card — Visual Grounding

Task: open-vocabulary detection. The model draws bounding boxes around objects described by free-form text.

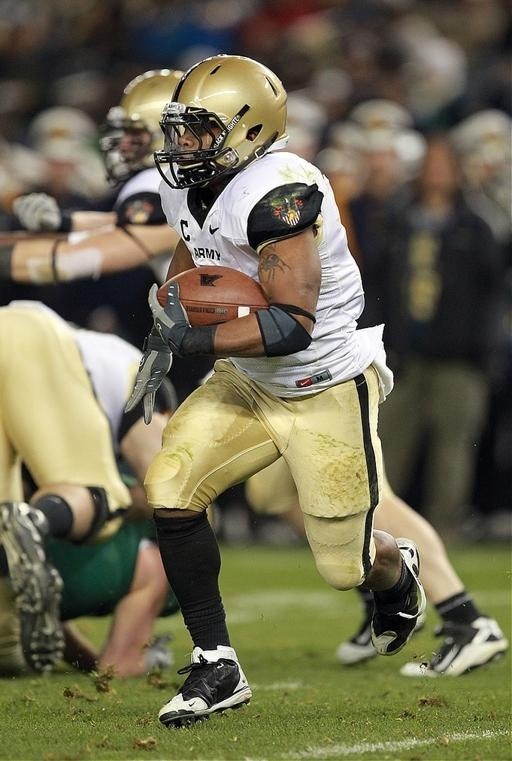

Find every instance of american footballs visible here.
[157,266,269,327]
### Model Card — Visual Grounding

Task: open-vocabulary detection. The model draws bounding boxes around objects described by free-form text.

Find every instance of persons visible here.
[0,66,510,680]
[2,1,510,551]
[124,55,426,726]
[33,521,180,678]
[0,295,181,674]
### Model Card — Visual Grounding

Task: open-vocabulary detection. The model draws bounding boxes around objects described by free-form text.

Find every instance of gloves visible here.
[149,281,216,358]
[125,325,172,425]
[13,192,71,233]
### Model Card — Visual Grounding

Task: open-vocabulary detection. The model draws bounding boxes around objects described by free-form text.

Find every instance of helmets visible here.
[98,54,289,189]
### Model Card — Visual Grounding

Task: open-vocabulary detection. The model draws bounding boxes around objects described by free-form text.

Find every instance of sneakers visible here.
[0,502,65,674]
[336,538,508,677]
[158,645,251,727]
